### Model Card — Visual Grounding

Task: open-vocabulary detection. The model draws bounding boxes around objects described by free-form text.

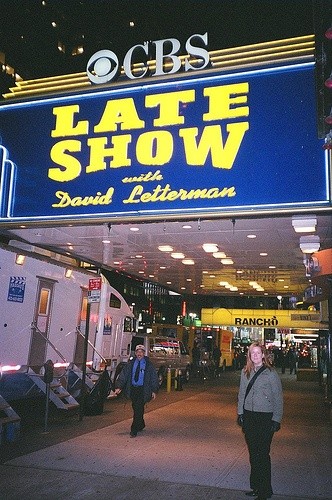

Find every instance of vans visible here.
[130,333,190,389]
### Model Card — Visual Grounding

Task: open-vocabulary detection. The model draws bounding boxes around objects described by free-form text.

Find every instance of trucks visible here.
[1,241,135,402]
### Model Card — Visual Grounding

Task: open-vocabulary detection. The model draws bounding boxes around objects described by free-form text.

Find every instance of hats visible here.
[136,345,145,351]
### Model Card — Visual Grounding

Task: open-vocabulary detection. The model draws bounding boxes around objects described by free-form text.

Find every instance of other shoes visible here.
[245,489,273,500]
[140,420,145,431]
[130,431,137,438]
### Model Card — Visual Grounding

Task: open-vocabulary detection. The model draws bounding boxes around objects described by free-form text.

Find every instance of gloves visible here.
[269,421,280,432]
[238,415,243,426]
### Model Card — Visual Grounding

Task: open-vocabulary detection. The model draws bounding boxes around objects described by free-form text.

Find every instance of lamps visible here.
[299,243,320,254]
[292,219,317,233]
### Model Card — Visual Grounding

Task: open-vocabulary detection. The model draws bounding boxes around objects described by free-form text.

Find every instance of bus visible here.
[151,323,236,374]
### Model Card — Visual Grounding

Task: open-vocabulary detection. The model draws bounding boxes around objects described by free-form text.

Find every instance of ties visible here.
[134,361,140,382]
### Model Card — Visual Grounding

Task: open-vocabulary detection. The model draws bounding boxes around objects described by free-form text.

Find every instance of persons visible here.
[237,342,283,500]
[298,342,311,368]
[114,345,159,438]
[231,345,298,375]
[192,342,201,366]
[212,345,221,367]
